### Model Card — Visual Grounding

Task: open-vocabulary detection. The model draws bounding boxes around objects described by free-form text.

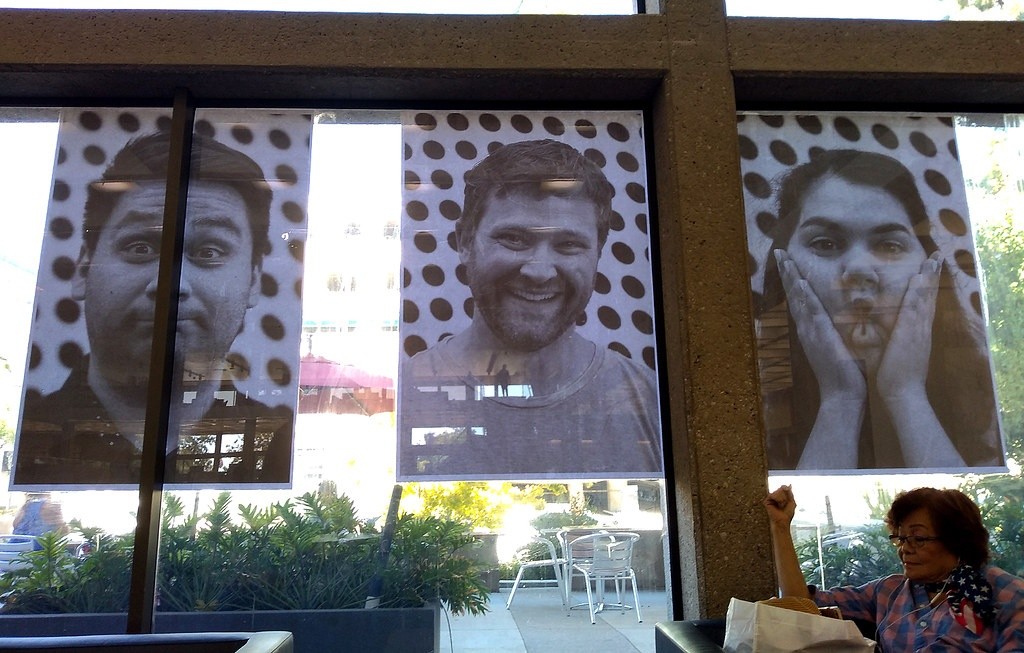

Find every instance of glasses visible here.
[889,534,940,547]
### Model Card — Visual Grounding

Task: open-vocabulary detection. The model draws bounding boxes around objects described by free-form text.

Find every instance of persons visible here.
[14,132,293,485]
[764,484,1024,653]
[400,139,664,476]
[757,149,1005,470]
[497,364,514,396]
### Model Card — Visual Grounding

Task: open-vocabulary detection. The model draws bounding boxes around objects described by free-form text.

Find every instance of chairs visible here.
[506,529,642,623]
[0,534,34,574]
